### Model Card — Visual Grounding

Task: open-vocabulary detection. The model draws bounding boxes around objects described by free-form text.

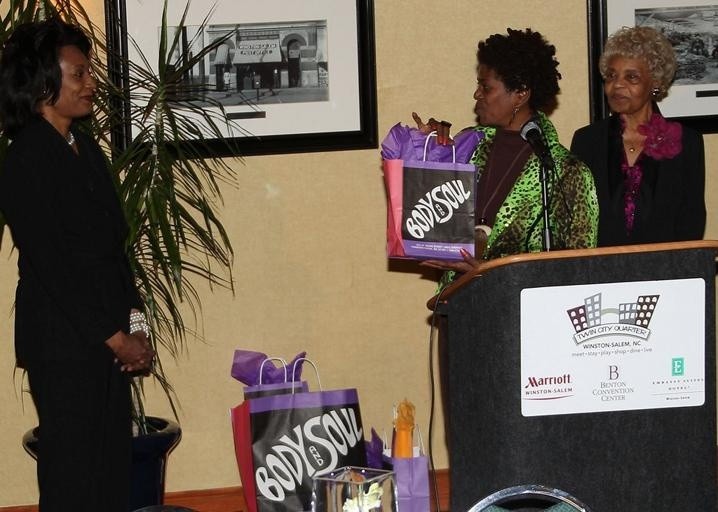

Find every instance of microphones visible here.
[521,118,556,171]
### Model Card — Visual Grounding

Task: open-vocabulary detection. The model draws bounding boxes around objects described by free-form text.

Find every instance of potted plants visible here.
[0,1,266,512]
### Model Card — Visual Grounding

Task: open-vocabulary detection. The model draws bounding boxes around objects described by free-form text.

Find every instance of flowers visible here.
[636,113,683,160]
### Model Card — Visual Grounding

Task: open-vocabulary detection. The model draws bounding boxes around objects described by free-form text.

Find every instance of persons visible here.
[412,25,600,294]
[690,33,704,54]
[570,29,706,246]
[1,16,156,511]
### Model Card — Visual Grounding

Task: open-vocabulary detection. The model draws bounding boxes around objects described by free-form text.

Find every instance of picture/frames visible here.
[586,1,718,135]
[104,0,379,164]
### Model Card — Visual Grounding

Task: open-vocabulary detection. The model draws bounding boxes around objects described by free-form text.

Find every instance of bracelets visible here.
[129,311,150,338]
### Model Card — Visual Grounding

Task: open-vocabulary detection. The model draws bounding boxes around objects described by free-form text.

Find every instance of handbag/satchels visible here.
[230,357,430,512]
[383,131,477,262]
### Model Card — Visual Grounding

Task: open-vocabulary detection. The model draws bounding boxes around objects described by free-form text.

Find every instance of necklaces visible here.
[622,140,641,152]
[68,131,75,147]
